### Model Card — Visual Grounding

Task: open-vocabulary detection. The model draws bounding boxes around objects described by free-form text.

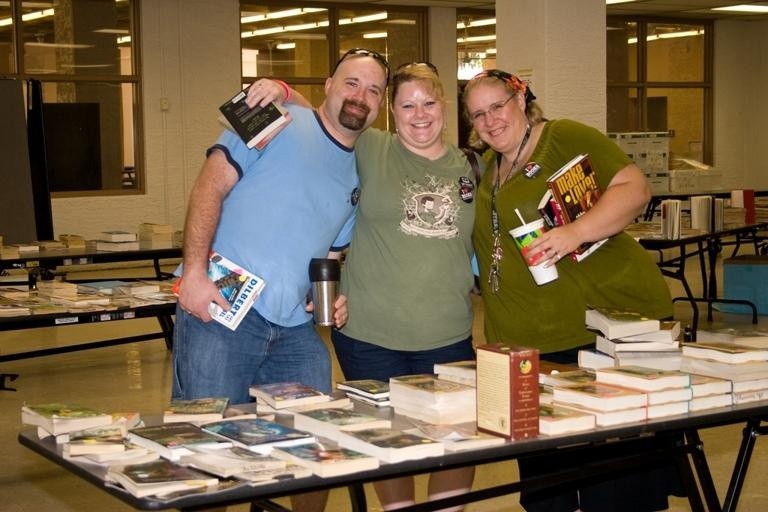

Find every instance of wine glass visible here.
[259,83,263,89]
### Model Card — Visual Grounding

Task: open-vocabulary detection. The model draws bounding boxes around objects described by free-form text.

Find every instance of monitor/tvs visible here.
[171,249,265,332]
[0,221,183,319]
[218,83,287,150]
[623,189,768,241]
[537,154,609,264]
[218,101,294,153]
[207,260,249,308]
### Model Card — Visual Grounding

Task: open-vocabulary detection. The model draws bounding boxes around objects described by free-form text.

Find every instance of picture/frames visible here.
[632,208,766,337]
[0,278,181,363]
[1,231,185,277]
[19,325,767,512]
[641,190,767,221]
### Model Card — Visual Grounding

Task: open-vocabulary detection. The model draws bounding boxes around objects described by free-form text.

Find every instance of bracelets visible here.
[277,79,292,102]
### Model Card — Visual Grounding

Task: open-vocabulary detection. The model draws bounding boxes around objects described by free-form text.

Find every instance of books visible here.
[338,428,444,463]
[22,401,382,502]
[207,260,249,308]
[537,154,609,264]
[218,101,294,153]
[248,381,354,415]
[623,189,768,241]
[294,411,391,442]
[218,83,287,150]
[337,305,767,452]
[0,221,183,319]
[171,249,265,332]
[606,128,724,196]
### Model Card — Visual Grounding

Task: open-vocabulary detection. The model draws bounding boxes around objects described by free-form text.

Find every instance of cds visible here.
[489,234,503,295]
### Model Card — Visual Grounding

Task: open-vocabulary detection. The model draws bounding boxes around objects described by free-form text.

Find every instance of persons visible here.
[170,48,393,512]
[461,69,690,512]
[244,61,488,512]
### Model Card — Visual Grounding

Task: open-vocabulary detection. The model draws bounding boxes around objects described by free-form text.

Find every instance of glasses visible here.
[454,13,481,79]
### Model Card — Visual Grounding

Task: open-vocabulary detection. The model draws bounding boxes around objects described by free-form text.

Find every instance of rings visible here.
[259,83,263,89]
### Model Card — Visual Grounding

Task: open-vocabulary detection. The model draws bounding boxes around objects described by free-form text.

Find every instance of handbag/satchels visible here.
[277,79,292,102]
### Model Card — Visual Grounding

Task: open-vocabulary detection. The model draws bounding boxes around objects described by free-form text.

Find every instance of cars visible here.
[508,216,560,287]
[307,257,341,328]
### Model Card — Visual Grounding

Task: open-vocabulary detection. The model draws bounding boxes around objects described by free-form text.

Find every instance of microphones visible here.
[720,255,766,316]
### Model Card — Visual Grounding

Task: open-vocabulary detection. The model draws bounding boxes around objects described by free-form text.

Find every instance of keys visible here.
[489,234,503,295]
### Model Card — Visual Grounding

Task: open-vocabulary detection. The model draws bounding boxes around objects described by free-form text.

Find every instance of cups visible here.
[397,61,439,77]
[468,90,519,122]
[330,47,391,85]
